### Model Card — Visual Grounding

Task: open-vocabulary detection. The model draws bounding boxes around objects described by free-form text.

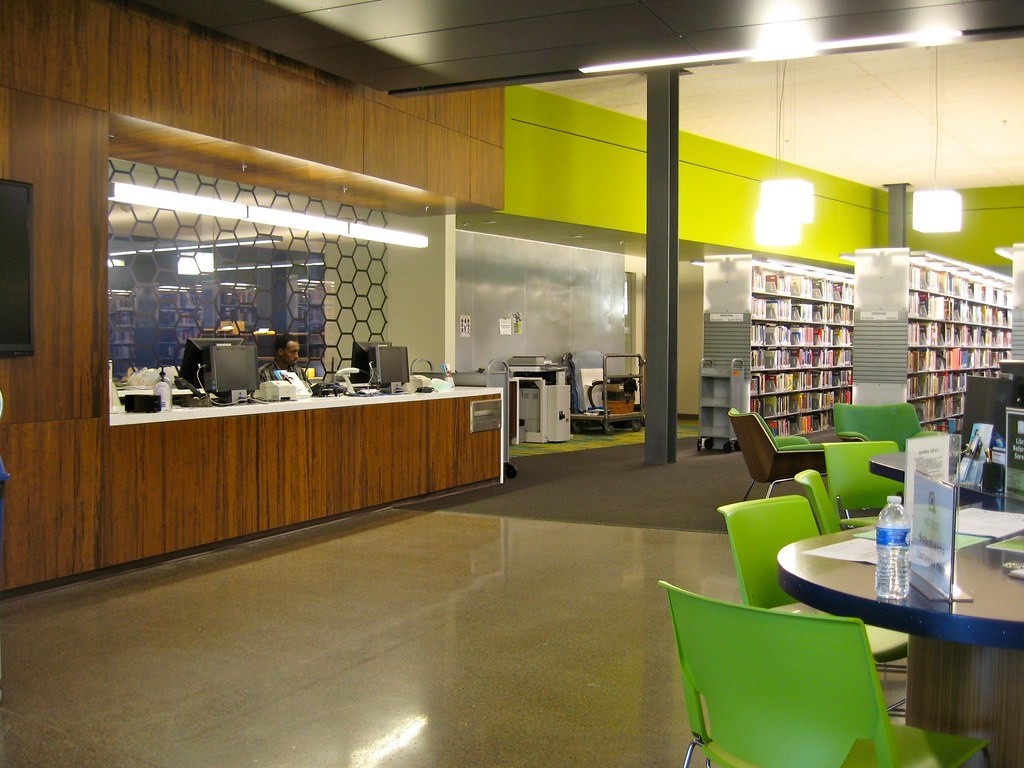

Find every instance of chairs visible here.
[821,441,905,527]
[793,469,842,535]
[832,402,946,452]
[727,408,854,530]
[656,580,993,768]
[718,494,908,713]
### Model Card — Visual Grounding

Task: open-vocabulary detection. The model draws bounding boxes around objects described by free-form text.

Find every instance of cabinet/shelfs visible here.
[161,289,201,367]
[839,248,1013,437]
[298,286,324,358]
[220,289,256,346]
[109,295,136,375]
[702,253,854,437]
[698,357,744,452]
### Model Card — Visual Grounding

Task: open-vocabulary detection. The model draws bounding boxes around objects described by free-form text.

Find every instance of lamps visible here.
[109,182,430,249]
[912,47,964,234]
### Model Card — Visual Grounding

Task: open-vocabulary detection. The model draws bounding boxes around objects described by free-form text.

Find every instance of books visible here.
[749,266,854,437]
[908,267,1013,434]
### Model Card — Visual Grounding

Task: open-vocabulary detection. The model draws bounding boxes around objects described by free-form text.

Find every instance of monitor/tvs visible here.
[179,338,260,406]
[349,340,410,395]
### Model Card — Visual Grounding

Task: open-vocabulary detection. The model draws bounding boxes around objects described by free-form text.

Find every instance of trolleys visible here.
[570,352,645,436]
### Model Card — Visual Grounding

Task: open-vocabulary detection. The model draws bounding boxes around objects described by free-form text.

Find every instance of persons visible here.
[258,333,310,384]
[966,429,980,455]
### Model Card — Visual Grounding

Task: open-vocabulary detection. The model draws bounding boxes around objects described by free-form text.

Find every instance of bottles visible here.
[875,495,912,598]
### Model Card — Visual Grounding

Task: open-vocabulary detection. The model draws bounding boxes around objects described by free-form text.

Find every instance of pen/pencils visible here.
[984,447,991,463]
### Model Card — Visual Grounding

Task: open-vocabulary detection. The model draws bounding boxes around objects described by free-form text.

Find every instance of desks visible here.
[776,452,1024,768]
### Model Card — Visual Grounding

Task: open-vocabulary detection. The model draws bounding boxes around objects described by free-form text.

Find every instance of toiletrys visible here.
[152,365,173,412]
